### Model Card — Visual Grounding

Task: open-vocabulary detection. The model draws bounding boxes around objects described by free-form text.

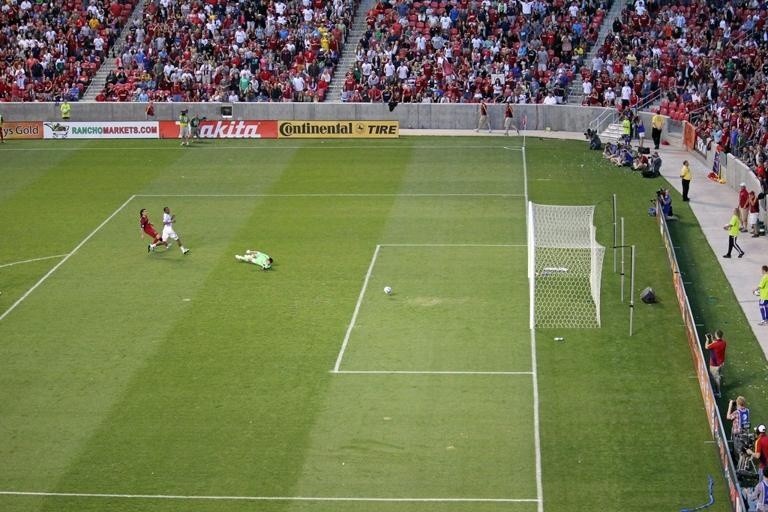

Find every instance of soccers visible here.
[384,286,391,293]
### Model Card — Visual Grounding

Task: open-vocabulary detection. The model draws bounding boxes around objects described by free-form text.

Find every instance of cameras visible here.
[648,155,655,159]
[707,335,711,338]
[728,398,737,406]
[584,133,590,135]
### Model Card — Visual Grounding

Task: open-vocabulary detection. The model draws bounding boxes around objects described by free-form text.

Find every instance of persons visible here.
[148,206,189,254]
[753,265,768,326]
[704,328,768,511]
[599,142,767,258]
[0,2,768,147]
[140,209,173,248]
[235,249,273,270]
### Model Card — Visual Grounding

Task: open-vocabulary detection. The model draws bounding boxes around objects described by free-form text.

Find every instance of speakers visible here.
[640,286,655,303]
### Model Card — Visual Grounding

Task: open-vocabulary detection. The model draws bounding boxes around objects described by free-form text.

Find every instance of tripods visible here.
[736,453,757,474]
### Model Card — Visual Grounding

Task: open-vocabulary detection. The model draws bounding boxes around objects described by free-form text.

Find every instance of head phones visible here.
[757,424,766,435]
